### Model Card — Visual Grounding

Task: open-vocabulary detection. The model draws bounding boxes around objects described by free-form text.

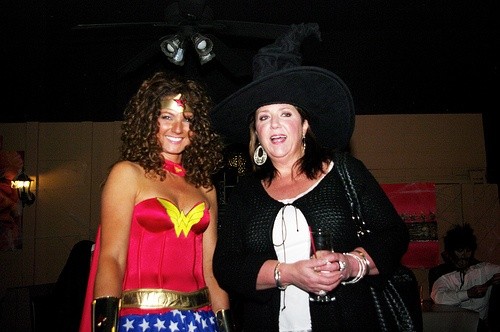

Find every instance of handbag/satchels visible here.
[335,156,423,332]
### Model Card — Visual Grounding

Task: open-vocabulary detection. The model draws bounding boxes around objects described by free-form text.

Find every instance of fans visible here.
[72,0,304,67]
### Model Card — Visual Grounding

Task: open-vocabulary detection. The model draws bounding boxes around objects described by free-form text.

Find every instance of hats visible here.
[210,66,354,178]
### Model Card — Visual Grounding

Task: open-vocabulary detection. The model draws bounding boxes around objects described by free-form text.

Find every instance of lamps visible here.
[14,151,35,205]
[161,32,216,65]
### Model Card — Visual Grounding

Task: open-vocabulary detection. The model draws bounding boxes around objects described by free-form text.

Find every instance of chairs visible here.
[29,240,94,332]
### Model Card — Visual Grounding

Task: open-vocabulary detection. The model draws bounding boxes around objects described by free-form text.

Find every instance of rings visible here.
[320,290,326,296]
[339,261,346,272]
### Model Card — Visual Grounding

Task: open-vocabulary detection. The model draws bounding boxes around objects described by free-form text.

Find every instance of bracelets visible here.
[355,250,370,276]
[342,253,365,286]
[274,260,287,290]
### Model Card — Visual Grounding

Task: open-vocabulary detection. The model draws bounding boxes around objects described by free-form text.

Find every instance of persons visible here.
[429,226,500,332]
[212,68,423,332]
[80,71,234,332]
